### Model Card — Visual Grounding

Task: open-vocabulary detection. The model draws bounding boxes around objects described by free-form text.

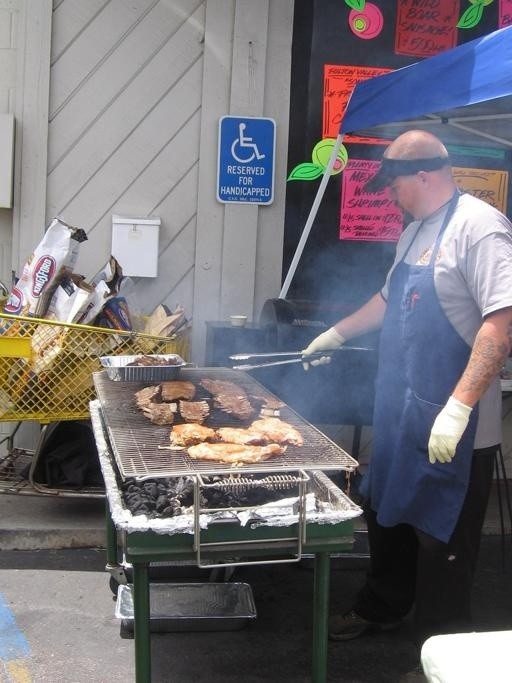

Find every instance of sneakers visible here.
[328,594,404,642]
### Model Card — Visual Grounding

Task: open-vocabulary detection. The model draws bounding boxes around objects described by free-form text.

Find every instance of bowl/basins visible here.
[229,315,248,327]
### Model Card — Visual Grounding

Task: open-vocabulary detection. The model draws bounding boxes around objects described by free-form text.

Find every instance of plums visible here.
[346,0,384,38]
[312,136,348,176]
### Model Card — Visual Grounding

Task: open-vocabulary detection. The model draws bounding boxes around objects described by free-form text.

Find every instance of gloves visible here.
[428,396,473,464]
[301,326,346,371]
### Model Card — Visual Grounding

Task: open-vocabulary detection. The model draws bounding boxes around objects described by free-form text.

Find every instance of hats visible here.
[359,155,455,193]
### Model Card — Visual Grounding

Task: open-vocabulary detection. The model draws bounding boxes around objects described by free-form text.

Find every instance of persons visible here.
[302,130,512,642]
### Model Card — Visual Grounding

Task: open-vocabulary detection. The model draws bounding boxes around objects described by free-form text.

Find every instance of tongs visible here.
[228,350,335,371]
